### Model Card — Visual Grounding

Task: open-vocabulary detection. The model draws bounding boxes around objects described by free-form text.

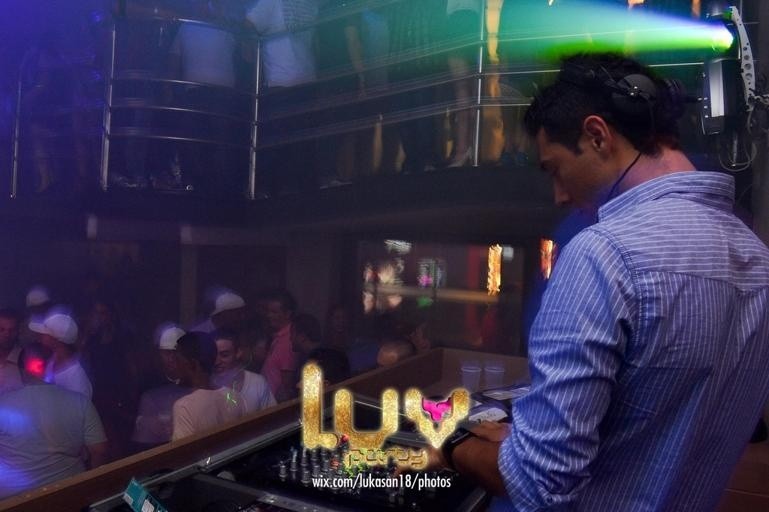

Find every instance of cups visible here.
[483,365,506,390]
[461,365,480,393]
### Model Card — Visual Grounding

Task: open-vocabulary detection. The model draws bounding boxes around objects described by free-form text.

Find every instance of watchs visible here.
[440,427,478,466]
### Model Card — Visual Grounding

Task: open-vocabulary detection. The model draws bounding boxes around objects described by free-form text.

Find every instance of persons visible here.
[0,280,452,503]
[393,49,769,512]
[0,0,709,196]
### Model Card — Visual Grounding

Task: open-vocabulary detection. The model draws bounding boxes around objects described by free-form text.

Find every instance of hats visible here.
[160,328,185,350]
[28,314,79,345]
[26,290,49,306]
[210,294,245,315]
